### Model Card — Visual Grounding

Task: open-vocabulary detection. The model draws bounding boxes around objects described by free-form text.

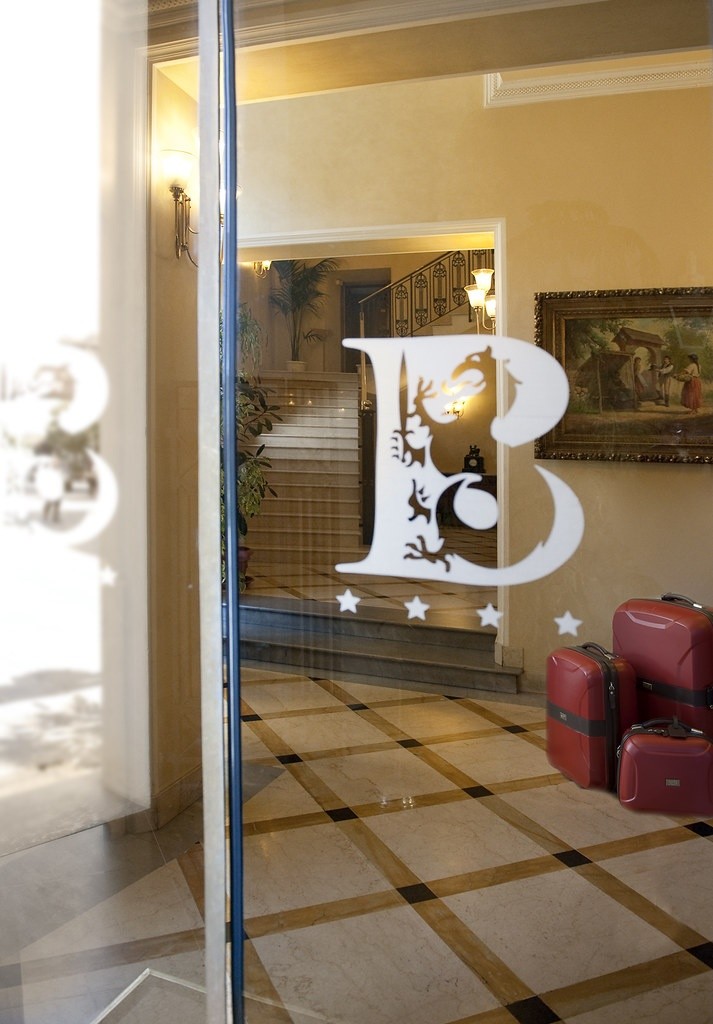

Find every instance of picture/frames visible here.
[534,284,713,464]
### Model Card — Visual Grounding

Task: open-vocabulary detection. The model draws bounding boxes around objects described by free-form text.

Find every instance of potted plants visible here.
[221,378,281,590]
[267,258,338,372]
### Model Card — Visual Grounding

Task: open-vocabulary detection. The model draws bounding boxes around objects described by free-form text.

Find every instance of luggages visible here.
[546,641,635,789]
[611,593,713,735]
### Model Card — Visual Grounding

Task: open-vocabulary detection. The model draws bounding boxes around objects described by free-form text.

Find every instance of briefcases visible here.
[616,719,713,816]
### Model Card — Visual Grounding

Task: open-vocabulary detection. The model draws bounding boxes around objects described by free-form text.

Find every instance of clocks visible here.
[439,381,464,415]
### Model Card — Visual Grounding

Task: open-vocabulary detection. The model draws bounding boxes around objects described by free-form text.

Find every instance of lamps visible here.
[161,150,239,266]
[463,262,498,339]
[251,260,274,278]
